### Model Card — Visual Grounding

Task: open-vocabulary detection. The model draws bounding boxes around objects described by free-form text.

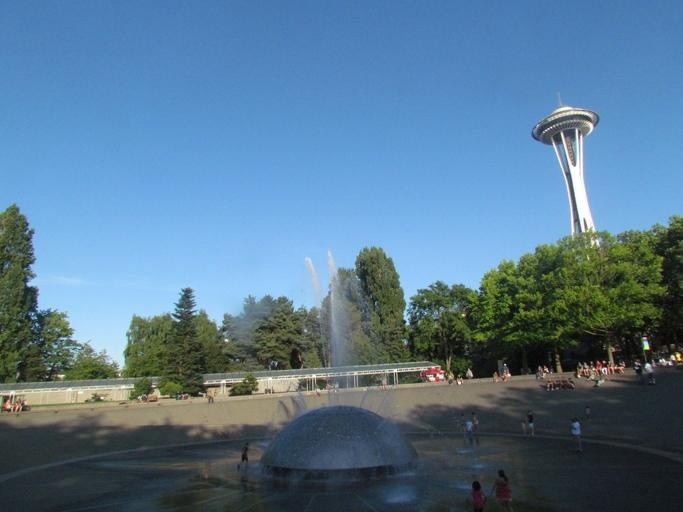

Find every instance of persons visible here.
[488,470,515,512]
[435,349,683,454]
[133,387,215,404]
[315,374,388,399]
[236,442,250,471]
[3,397,32,413]
[466,479,487,511]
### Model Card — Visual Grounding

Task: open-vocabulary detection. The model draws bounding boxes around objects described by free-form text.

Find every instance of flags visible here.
[641,337,650,351]
